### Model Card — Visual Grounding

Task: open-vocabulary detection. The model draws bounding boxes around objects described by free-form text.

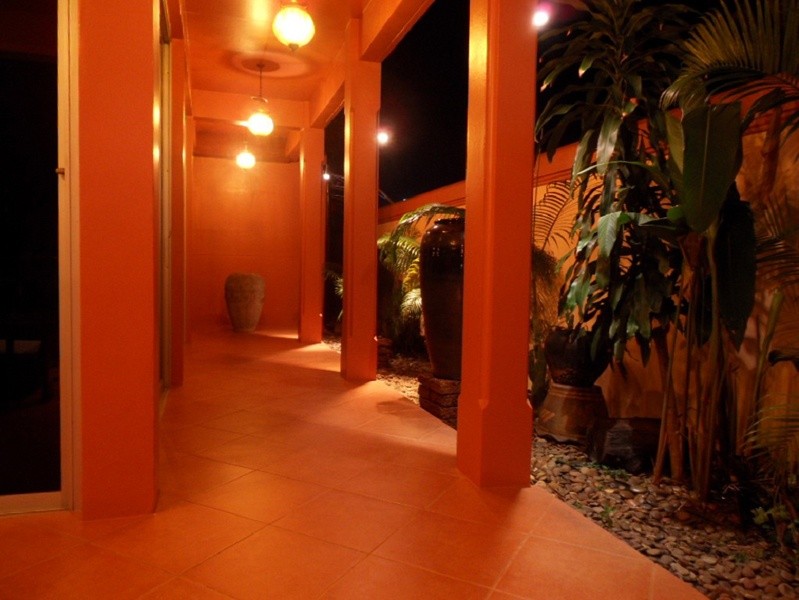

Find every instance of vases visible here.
[225,272,263,332]
[418,219,465,378]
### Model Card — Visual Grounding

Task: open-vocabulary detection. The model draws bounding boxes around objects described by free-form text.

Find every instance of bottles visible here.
[225,272,264,334]
[419,218,465,379]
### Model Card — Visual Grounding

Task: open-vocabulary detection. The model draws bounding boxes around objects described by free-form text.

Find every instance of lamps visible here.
[247,64,274,135]
[236,138,255,170]
[272,0,315,53]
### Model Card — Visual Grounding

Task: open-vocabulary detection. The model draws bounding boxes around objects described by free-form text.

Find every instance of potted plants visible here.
[536,0,687,386]
